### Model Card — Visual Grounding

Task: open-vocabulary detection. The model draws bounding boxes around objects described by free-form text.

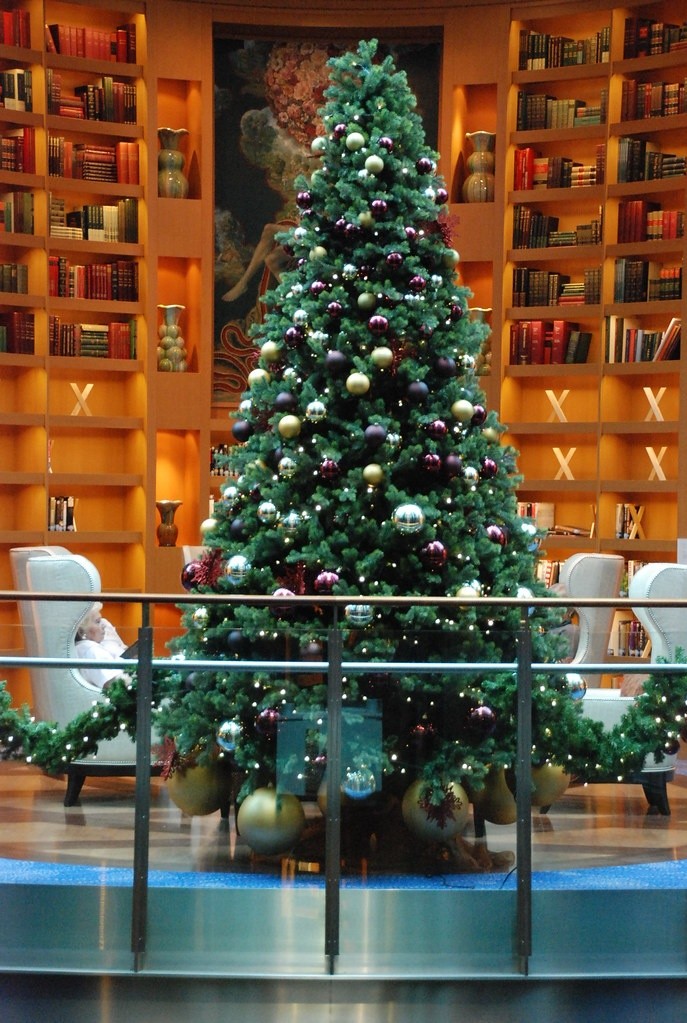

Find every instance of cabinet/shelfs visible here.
[0,0,214,715]
[437,0,687,689]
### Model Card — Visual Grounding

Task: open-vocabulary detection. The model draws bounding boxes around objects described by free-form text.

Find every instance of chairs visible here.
[25,554,174,808]
[539,562,687,817]
[7,544,73,782]
[558,552,625,688]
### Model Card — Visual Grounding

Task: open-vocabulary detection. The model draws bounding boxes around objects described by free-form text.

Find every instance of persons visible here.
[620,673,650,697]
[75,600,132,690]
[542,582,580,661]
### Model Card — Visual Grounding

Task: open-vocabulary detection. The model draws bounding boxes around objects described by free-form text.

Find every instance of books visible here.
[0,9,139,360]
[49,495,79,531]
[510,15,687,365]
[517,501,591,588]
[616,504,641,539]
[619,559,657,656]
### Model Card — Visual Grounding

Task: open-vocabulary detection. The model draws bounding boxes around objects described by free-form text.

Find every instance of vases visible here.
[155,500,184,548]
[462,129,496,203]
[157,303,189,373]
[158,127,190,199]
